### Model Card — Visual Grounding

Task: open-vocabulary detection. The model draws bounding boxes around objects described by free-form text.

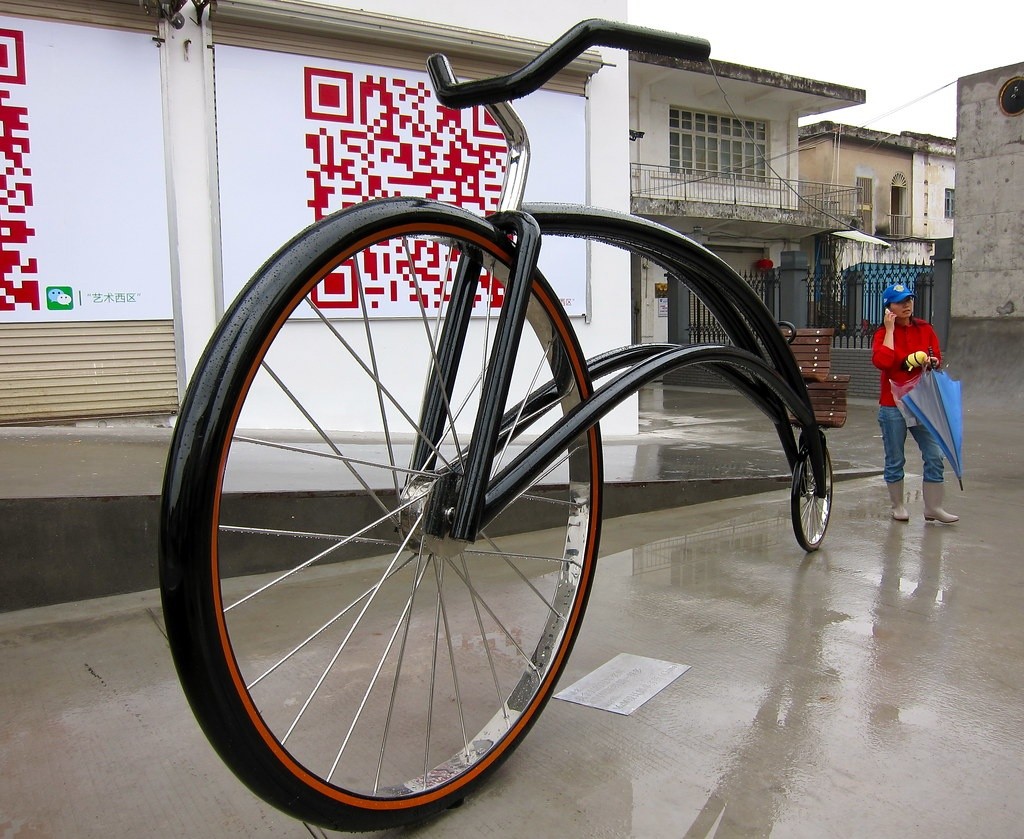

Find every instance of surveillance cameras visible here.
[629,128,644,139]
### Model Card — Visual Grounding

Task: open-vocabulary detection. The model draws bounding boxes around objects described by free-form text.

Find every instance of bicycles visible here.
[158,18,834,834]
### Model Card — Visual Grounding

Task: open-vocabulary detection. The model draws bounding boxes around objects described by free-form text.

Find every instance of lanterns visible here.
[754,258,774,273]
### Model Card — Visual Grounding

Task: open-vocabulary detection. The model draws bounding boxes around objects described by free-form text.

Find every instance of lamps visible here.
[138,0,210,30]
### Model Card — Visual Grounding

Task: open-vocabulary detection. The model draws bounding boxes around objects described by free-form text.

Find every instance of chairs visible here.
[789,374,852,426]
[782,328,835,382]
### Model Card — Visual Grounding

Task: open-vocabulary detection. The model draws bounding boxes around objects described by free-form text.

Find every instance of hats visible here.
[882,284,915,306]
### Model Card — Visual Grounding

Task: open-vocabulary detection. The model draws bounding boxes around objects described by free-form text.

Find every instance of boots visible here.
[922,481,959,523]
[886,479,909,520]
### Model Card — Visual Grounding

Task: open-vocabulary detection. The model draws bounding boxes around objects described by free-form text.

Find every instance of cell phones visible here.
[885,307,895,322]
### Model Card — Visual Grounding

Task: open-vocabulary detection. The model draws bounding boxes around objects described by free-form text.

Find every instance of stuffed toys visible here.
[900,351,929,373]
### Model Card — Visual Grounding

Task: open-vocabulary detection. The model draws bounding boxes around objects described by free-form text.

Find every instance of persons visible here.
[872,284,959,523]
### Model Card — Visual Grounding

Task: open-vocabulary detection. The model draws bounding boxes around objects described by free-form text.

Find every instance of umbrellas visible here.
[901,347,964,491]
